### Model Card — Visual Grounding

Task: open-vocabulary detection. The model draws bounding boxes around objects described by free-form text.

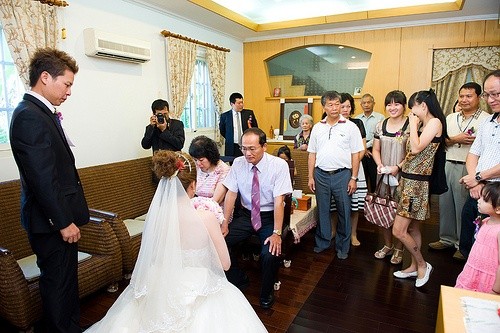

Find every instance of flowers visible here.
[188,196,226,225]
[473,215,482,234]
[176,161,186,171]
[468,127,477,135]
[57,113,65,124]
[393,131,404,147]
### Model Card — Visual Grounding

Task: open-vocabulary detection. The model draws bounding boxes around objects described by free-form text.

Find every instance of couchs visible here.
[77,155,160,280]
[274,148,316,194]
[0,179,123,333]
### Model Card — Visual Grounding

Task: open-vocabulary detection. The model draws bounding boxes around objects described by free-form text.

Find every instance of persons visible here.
[392,89,447,286]
[141,99,185,190]
[189,136,234,225]
[293,114,314,151]
[330,92,368,246]
[459,69,500,260]
[83,151,268,333]
[219,92,258,166]
[454,181,500,295]
[428,82,491,260]
[10,47,90,333]
[277,145,298,190]
[453,100,461,114]
[372,90,410,264]
[219,127,293,309]
[307,91,365,263]
[353,94,386,193]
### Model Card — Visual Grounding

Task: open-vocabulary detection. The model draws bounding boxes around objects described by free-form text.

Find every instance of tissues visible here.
[292,190,311,211]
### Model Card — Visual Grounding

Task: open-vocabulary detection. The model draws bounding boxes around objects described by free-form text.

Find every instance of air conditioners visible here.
[83,27,152,65]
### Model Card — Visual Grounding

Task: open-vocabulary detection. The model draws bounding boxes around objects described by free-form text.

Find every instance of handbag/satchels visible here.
[429,152,448,195]
[364,173,398,230]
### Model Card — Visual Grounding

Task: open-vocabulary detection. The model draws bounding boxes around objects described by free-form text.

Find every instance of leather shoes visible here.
[259,295,275,309]
[393,271,418,278]
[415,262,432,287]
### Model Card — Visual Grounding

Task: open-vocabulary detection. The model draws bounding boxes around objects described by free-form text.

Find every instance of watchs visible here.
[273,230,283,236]
[475,172,483,183]
[351,176,359,182]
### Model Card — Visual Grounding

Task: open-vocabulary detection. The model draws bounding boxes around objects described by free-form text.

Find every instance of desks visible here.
[248,194,318,247]
[262,140,296,156]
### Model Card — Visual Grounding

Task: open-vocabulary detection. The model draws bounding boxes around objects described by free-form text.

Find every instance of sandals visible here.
[390,246,405,264]
[375,244,394,259]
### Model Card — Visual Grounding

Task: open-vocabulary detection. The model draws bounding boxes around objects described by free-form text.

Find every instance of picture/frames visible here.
[279,97,314,140]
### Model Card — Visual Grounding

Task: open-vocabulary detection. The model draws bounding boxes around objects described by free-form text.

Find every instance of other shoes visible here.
[242,254,249,260]
[314,247,323,253]
[453,250,464,259]
[352,235,360,245]
[337,253,348,259]
[253,253,259,261]
[428,241,447,249]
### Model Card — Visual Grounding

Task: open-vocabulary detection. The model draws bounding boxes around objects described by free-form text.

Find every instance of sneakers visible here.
[274,281,281,290]
[283,260,291,268]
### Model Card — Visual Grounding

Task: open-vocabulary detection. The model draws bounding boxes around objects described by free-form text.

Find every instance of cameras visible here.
[156,113,166,124]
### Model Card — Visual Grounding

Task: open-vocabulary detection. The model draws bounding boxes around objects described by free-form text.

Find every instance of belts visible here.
[446,160,466,165]
[316,166,351,175]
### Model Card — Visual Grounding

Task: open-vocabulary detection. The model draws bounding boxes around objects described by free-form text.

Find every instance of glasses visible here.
[239,146,261,151]
[483,91,500,98]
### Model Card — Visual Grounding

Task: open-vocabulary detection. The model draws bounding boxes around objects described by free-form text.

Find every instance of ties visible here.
[251,165,261,231]
[235,112,241,147]
[53,113,61,129]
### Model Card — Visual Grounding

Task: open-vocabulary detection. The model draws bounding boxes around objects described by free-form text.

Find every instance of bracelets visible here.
[396,164,402,170]
[492,289,500,295]
